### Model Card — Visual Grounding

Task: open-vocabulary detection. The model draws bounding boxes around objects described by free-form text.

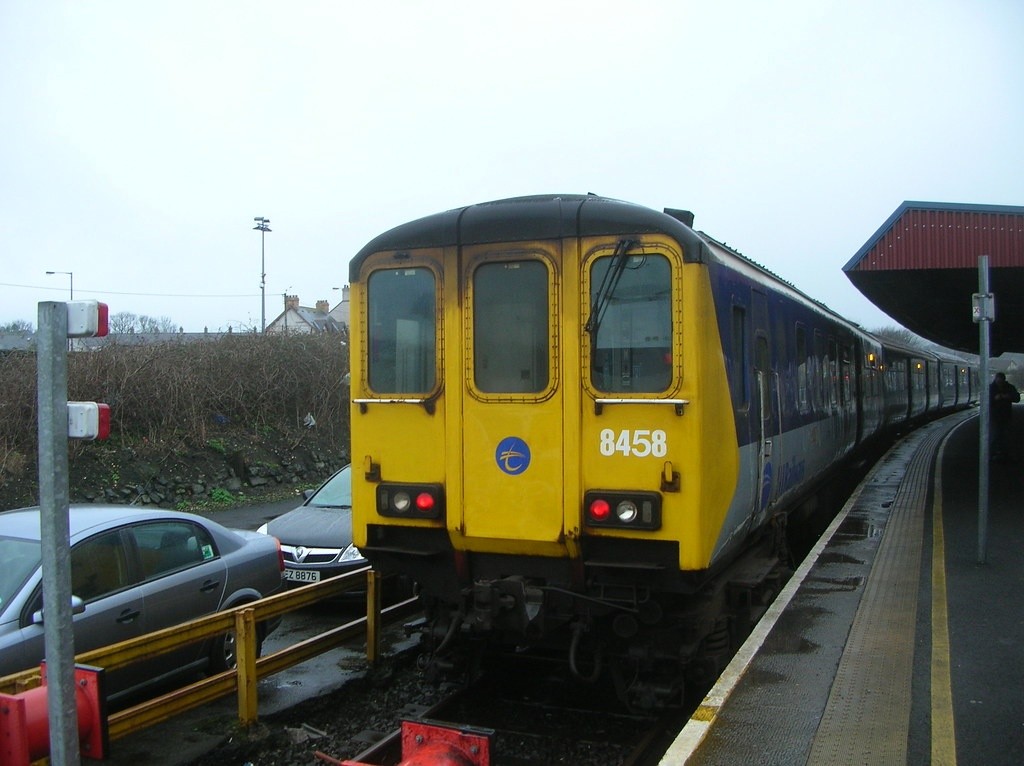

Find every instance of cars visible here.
[253,461,370,598]
[0,504,283,707]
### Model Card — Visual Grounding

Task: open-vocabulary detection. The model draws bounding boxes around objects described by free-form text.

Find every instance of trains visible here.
[347,193,983,766]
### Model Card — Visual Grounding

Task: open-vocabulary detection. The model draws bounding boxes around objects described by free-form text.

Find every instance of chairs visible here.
[146,527,195,577]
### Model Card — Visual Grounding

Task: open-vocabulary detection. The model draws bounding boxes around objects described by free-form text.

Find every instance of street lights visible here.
[47,271,73,354]
[254,217,272,335]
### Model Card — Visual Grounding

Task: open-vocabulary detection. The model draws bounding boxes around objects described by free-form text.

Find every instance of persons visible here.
[989,372,1020,460]
